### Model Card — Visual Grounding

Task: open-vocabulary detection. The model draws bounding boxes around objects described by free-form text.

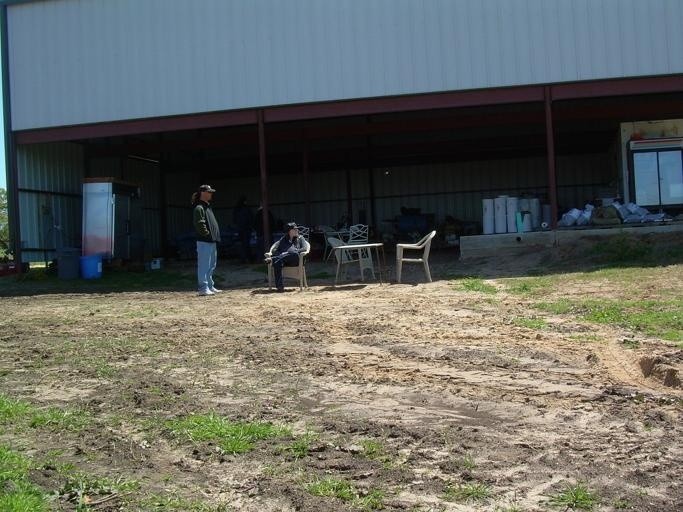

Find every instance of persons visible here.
[232,197,254,264]
[443,216,459,244]
[190,185,221,295]
[264,223,311,292]
[254,199,275,264]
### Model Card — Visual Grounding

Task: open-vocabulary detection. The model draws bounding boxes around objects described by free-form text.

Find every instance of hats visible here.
[199,185,216,193]
[287,223,300,232]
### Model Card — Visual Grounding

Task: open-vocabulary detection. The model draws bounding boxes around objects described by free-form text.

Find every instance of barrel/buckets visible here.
[80,255,103,280]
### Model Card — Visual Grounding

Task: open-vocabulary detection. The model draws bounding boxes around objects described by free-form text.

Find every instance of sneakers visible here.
[199,287,222,296]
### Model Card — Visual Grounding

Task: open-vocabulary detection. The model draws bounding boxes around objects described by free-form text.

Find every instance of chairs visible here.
[295,226,309,241]
[319,225,336,260]
[264,237,311,290]
[396,230,436,283]
[328,237,358,283]
[349,224,369,257]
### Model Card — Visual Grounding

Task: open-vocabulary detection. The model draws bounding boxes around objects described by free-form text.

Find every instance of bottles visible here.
[514,211,524,233]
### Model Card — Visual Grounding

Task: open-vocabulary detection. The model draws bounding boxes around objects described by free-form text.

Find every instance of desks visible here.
[332,243,386,287]
[327,231,350,260]
